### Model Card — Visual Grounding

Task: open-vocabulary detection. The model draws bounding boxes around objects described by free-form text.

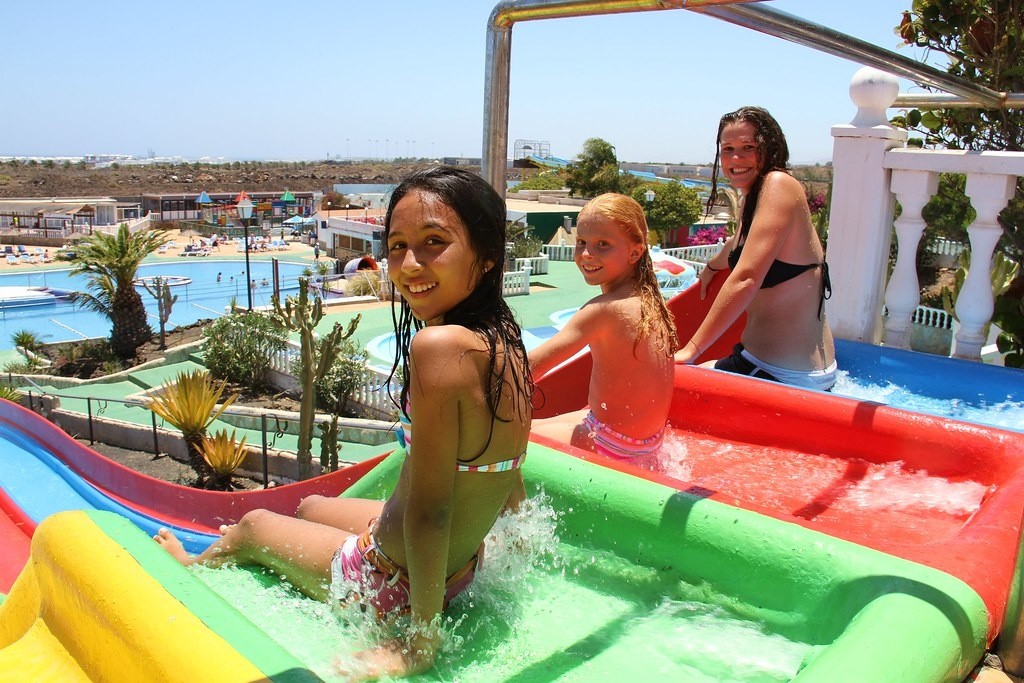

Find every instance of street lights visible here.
[644,190,655,227]
[365,207,368,223]
[233,197,258,312]
[328,202,331,217]
[346,204,349,220]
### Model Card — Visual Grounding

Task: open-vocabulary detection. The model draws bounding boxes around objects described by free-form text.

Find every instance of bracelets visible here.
[706,261,717,272]
[691,339,702,358]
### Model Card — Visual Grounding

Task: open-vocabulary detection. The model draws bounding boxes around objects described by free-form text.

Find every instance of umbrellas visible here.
[283,215,305,233]
[303,218,316,225]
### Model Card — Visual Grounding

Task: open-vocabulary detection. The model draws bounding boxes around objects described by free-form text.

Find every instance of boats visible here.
[132,275,194,288]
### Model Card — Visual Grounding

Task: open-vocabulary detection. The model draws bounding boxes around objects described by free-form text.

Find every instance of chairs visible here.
[180,234,228,257]
[159,239,179,253]
[233,235,291,253]
[61,244,77,260]
[0,244,52,264]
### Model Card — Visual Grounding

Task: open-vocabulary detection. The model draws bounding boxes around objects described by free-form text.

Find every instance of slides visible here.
[523,155,730,192]
[0,266,1024,683]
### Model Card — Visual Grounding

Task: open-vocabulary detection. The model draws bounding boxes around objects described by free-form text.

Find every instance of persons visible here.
[43,216,386,289]
[670,108,838,396]
[525,192,675,464]
[153,164,544,682]
